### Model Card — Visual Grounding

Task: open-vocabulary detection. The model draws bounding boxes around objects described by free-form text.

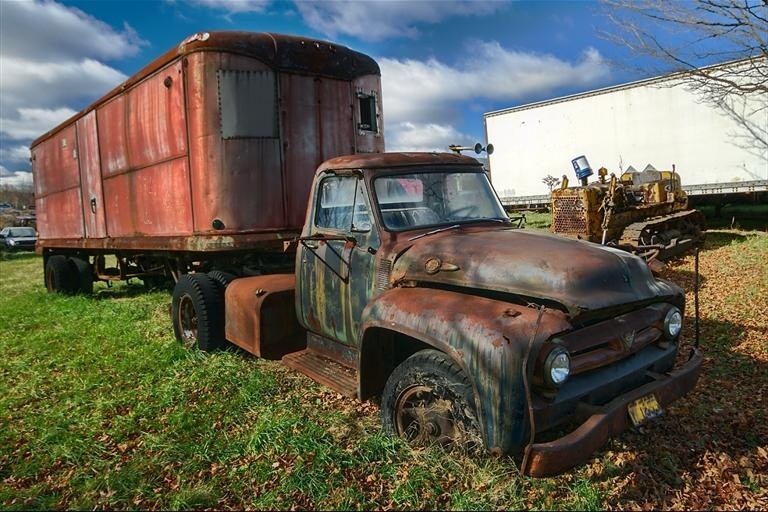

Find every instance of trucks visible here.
[29,30,704,478]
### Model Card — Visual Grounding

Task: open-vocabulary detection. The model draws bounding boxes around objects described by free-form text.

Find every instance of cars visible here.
[0,226,36,252]
[0,203,15,213]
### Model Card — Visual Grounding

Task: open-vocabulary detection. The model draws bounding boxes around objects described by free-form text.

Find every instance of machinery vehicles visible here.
[550,156,703,259]
